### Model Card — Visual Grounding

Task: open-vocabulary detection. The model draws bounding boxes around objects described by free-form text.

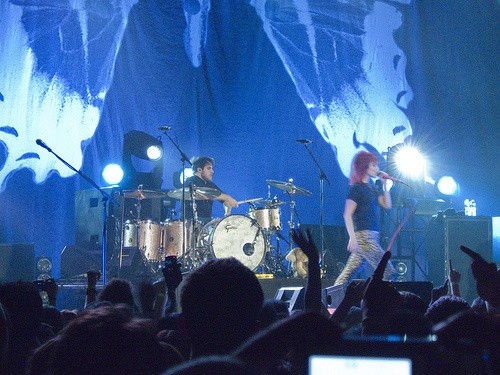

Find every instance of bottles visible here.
[464,199,469,215]
[467,200,476,216]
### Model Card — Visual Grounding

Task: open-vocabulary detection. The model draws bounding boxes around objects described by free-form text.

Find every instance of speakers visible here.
[108,246,147,282]
[393,281,435,310]
[273,287,330,316]
[0,244,35,283]
[60,246,99,279]
[430,216,492,307]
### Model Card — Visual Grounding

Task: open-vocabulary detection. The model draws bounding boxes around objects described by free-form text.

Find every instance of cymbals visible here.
[121,189,166,199]
[256,199,285,206]
[167,186,221,201]
[265,180,313,195]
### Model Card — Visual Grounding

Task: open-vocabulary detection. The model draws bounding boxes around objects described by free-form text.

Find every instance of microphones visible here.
[295,139,314,144]
[383,176,403,184]
[158,126,171,130]
[36,139,52,152]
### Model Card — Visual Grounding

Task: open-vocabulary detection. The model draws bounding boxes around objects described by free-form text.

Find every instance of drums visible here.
[248,206,281,230]
[123,219,161,264]
[197,213,266,272]
[162,218,204,260]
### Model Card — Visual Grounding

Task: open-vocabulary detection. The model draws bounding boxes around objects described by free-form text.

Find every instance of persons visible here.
[0,226,500,375]
[334,151,401,284]
[181,157,239,237]
